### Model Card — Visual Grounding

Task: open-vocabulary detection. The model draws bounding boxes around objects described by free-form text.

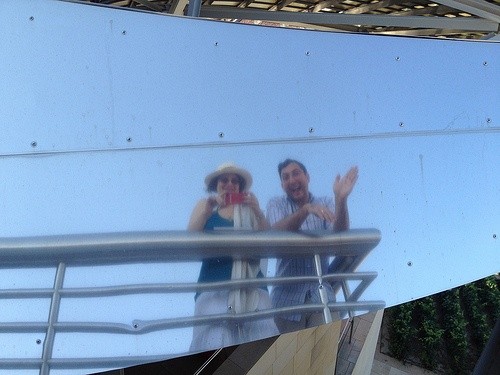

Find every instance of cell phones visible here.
[223,193,246,205]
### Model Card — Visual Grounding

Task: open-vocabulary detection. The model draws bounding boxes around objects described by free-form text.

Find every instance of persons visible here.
[188,162,281,354]
[266,158,359,334]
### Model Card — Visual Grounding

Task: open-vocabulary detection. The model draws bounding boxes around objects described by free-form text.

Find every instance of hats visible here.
[204,163,253,192]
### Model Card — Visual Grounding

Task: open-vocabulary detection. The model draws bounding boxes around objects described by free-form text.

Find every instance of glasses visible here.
[218,177,240,185]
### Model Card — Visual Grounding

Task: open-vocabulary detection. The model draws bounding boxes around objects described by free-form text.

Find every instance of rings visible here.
[321,210,323,214]
